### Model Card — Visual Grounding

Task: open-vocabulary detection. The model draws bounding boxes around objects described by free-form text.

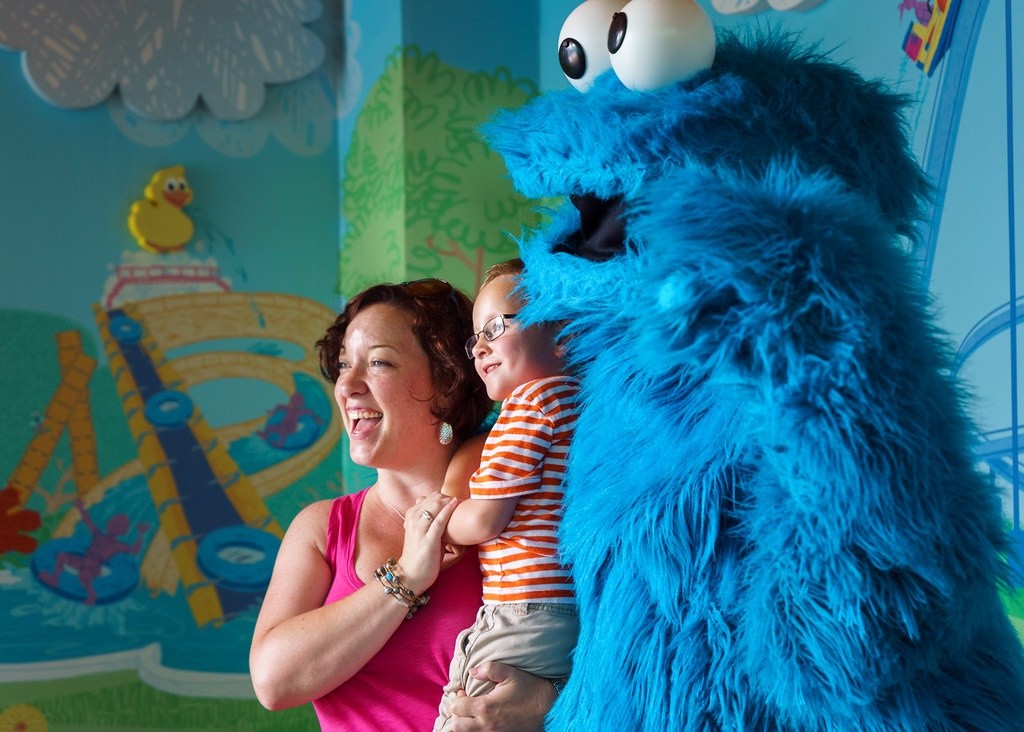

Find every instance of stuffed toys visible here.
[477,1,1024,732]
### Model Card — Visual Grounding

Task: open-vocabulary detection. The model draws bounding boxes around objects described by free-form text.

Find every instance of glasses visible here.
[464,313,560,360]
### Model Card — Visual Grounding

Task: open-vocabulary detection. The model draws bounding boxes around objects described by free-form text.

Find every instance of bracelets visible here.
[373,558,430,618]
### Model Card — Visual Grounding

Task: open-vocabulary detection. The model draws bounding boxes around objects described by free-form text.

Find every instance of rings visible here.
[422,509,433,522]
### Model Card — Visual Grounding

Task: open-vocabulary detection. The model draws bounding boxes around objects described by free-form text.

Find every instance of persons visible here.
[247,280,496,732]
[403,258,580,732]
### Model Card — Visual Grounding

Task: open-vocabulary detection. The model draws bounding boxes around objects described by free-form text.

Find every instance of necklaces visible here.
[375,482,405,520]
